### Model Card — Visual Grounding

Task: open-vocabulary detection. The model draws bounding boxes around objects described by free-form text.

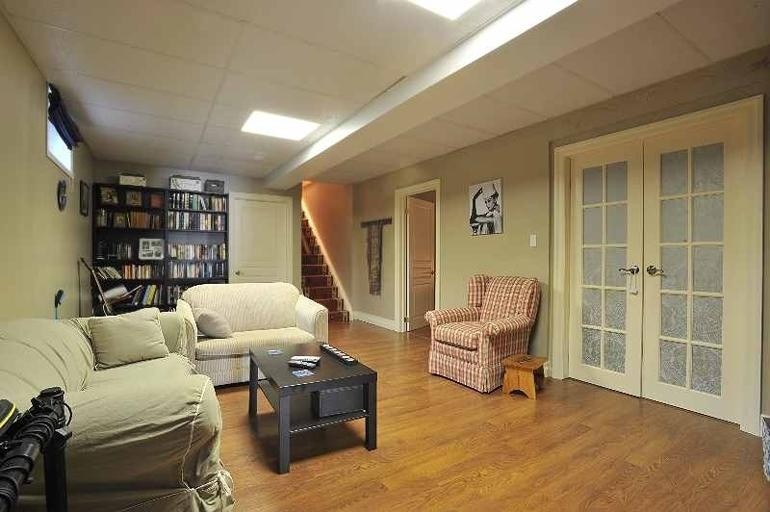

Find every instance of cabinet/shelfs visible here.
[93,183,229,316]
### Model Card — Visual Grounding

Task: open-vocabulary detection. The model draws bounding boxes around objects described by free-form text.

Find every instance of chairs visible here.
[425,275,541,393]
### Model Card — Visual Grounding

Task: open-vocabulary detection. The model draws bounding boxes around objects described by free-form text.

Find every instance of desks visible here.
[249,341,377,474]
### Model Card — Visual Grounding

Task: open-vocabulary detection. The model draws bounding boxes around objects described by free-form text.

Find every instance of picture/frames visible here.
[80,181,90,216]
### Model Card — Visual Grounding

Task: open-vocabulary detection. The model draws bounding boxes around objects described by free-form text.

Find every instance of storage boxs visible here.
[204,179,224,192]
[311,384,365,417]
[119,175,146,186]
[169,174,202,192]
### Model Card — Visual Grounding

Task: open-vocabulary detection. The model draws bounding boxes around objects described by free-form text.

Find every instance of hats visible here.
[482,184,497,198]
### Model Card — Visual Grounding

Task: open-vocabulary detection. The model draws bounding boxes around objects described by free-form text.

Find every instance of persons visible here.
[470,184,501,233]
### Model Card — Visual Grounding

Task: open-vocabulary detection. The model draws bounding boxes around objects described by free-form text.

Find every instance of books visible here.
[92,188,228,311]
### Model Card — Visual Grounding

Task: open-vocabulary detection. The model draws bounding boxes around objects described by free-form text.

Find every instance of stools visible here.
[500,354,547,400]
